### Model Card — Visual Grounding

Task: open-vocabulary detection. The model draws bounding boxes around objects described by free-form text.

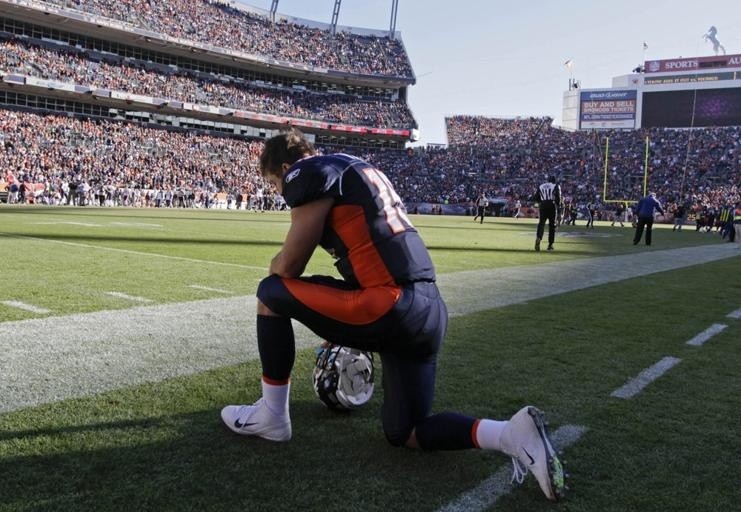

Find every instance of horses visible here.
[702,26,726,56]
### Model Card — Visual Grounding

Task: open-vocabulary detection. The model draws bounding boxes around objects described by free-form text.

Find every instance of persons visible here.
[535,176,561,250]
[221,132,571,502]
[633,193,664,245]
[409,115,740,241]
[0,0,418,212]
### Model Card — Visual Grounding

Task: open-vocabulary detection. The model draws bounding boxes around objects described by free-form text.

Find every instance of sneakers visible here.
[506,404,564,501]
[535,238,554,251]
[221,398,292,442]
